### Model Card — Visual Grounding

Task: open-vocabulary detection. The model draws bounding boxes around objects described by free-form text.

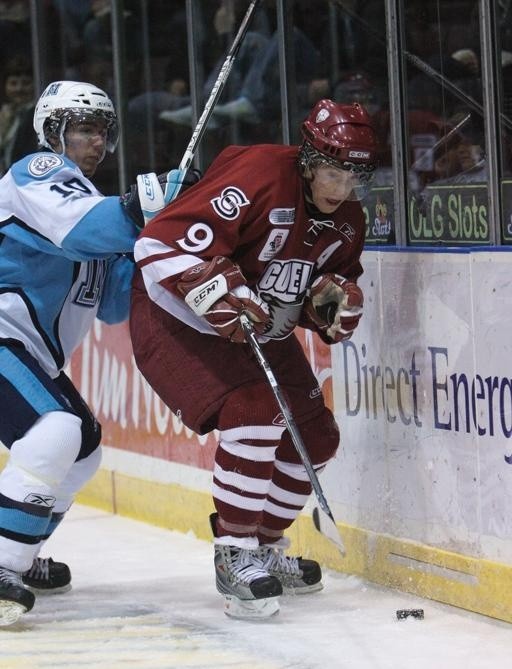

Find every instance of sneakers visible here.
[1,567,35,614]
[215,98,262,125]
[162,106,196,128]
[24,556,71,589]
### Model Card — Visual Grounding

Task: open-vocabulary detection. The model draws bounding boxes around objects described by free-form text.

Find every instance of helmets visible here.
[303,100,379,202]
[34,79,121,153]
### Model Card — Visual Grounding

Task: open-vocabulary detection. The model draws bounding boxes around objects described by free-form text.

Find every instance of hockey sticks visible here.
[240,307,344,553]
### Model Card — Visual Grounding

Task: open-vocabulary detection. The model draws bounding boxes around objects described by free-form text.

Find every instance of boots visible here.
[209,512,322,599]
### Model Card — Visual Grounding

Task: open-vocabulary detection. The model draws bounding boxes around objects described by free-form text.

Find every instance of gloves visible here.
[302,274,362,343]
[177,256,269,345]
[122,168,204,228]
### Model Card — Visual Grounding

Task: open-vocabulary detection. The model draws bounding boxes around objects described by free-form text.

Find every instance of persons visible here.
[124,97,379,601]
[1,1,512,185]
[0,81,203,613]
